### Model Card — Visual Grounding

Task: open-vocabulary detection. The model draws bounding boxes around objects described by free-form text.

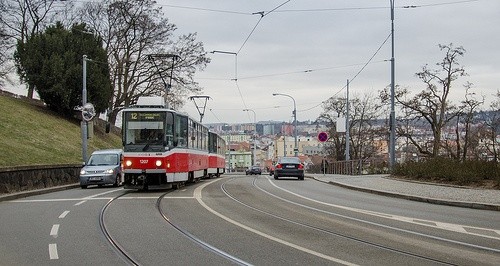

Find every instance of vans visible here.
[79,149,122,189]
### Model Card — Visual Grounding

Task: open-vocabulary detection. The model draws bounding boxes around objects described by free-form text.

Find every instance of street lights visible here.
[243,109,257,167]
[273,93,298,157]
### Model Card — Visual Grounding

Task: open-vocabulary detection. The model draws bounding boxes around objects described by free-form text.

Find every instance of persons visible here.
[321,156,329,174]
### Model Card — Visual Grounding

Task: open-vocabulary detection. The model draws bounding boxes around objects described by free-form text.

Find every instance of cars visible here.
[245,165,262,175]
[270,165,275,176]
[273,156,305,180]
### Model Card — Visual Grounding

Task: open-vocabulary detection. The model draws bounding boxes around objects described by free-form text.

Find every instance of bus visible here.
[119,95,226,192]
